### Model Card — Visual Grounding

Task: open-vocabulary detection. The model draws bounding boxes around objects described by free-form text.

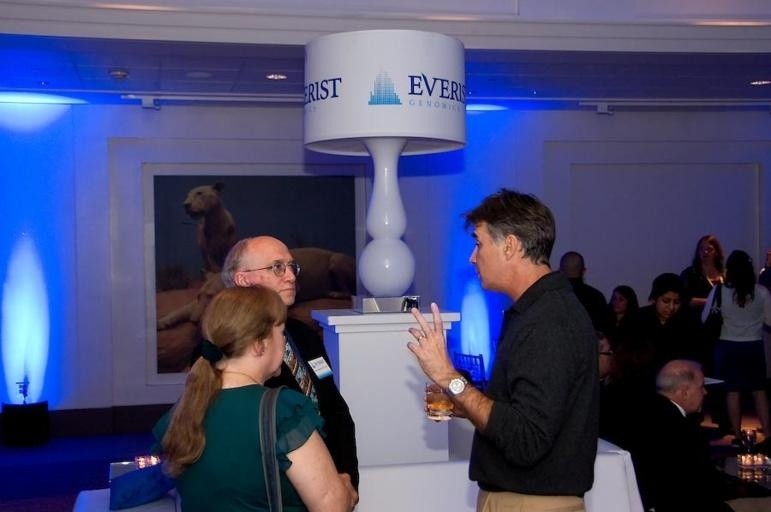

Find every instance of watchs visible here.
[446,375,468,398]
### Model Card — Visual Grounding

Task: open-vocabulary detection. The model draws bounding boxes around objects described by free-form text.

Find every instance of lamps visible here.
[302,29,464,296]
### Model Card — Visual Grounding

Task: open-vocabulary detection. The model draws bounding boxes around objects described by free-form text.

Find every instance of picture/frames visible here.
[139,163,368,385]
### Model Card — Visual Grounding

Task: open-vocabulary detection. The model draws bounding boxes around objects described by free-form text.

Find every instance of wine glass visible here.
[739,428,757,459]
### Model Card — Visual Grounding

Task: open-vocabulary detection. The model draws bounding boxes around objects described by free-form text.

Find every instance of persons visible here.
[407,188,600,512]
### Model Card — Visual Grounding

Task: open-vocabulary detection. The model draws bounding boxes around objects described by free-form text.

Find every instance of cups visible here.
[422,378,456,422]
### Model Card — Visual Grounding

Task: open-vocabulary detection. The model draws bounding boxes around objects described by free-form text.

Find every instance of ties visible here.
[280,330,322,417]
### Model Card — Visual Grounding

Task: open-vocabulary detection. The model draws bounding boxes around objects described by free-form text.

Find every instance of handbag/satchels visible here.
[704,284,723,336]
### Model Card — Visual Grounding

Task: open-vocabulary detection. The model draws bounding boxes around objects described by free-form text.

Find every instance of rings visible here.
[418,335,424,342]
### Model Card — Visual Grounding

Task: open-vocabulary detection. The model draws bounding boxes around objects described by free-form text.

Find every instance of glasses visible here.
[244,260,301,277]
